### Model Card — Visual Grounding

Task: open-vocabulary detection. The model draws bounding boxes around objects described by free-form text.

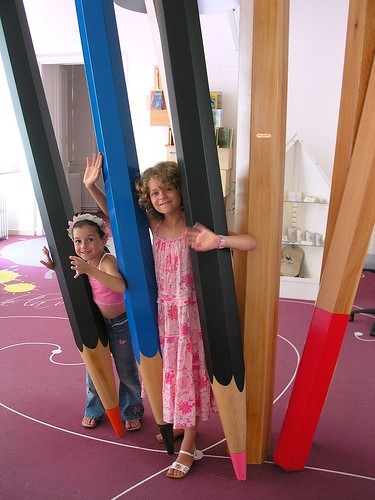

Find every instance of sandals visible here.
[81,416,97,428]
[155,429,184,442]
[125,419,141,431]
[165,446,197,479]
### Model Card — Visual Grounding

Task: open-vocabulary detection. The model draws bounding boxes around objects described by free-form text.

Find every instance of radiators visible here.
[0,193,9,240]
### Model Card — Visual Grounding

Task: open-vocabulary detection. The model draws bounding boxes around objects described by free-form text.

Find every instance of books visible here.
[150,91,234,148]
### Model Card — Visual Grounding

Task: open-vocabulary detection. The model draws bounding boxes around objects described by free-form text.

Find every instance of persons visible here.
[41,213,144,432]
[83,152,257,479]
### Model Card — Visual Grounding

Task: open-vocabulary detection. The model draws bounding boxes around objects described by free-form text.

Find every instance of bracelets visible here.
[217,235,225,249]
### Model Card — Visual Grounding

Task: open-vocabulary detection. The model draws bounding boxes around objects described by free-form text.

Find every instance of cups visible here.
[288,227,324,242]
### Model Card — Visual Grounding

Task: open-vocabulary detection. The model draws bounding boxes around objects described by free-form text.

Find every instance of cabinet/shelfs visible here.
[149,67,233,207]
[280,200,328,300]
